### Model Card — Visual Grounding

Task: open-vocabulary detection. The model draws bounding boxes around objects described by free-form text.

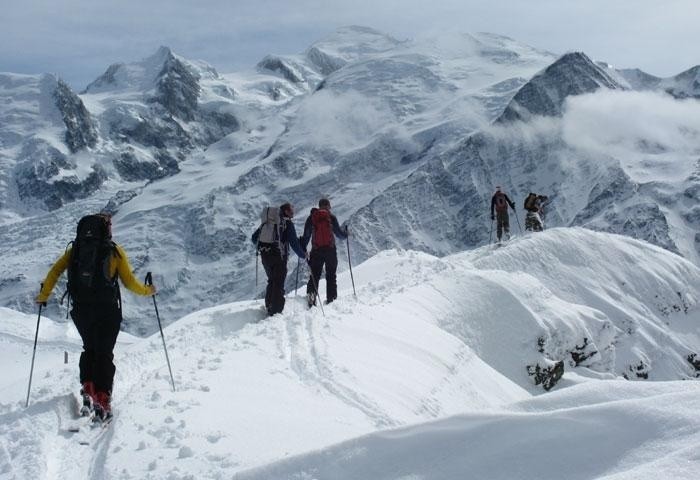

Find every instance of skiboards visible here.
[67,415,112,446]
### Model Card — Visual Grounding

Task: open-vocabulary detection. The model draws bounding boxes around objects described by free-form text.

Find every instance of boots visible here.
[307,291,316,305]
[80,382,112,420]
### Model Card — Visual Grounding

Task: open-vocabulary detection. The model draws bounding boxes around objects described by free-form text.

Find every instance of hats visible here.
[319,199,330,207]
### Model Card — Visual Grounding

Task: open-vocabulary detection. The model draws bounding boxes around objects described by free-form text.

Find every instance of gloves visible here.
[490,215,495,220]
[511,205,515,209]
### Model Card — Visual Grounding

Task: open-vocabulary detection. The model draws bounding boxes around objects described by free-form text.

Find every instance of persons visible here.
[523,192,549,231]
[491,183,516,241]
[297,197,351,310]
[35,214,158,423]
[252,201,308,319]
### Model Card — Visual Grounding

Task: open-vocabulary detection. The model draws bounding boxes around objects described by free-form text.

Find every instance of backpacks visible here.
[257,206,285,259]
[67,216,110,302]
[311,209,334,249]
[496,196,507,209]
[526,196,536,209]
